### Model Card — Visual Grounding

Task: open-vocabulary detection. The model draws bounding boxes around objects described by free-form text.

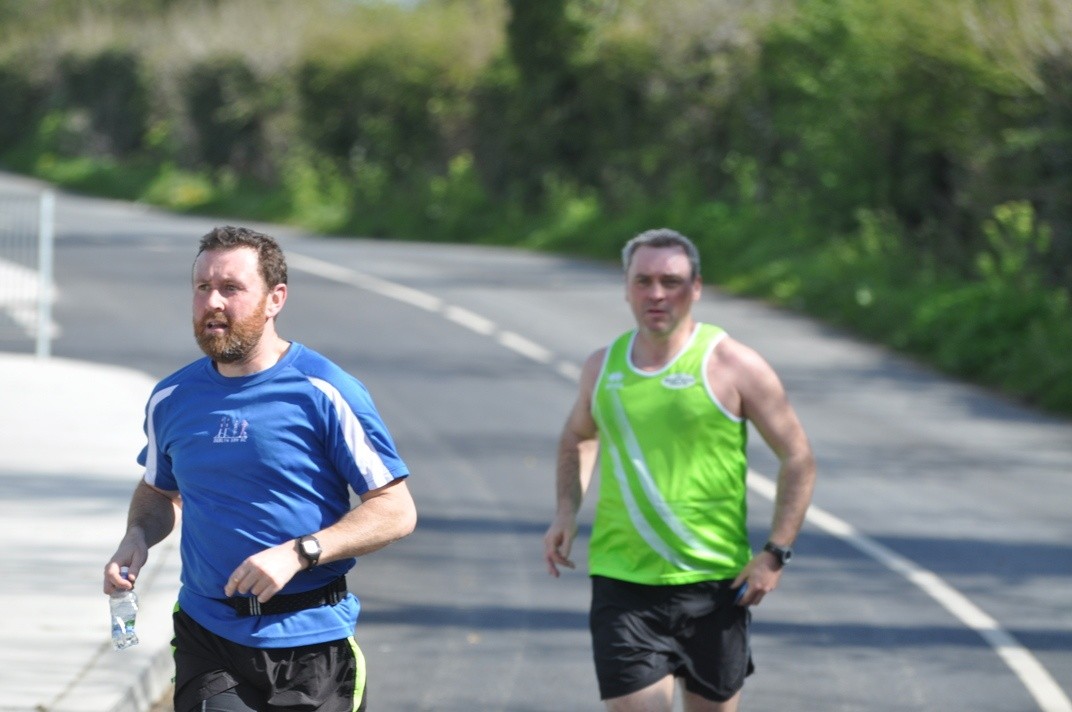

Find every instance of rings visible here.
[229,576,239,582]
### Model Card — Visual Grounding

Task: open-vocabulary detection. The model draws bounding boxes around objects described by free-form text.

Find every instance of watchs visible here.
[765,542,793,565]
[299,535,321,570]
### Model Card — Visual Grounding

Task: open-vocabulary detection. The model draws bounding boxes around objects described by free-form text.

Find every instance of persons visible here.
[545,229,814,712]
[104,227,418,712]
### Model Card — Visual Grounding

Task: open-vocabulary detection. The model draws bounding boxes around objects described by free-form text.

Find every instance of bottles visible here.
[110,567,139,651]
